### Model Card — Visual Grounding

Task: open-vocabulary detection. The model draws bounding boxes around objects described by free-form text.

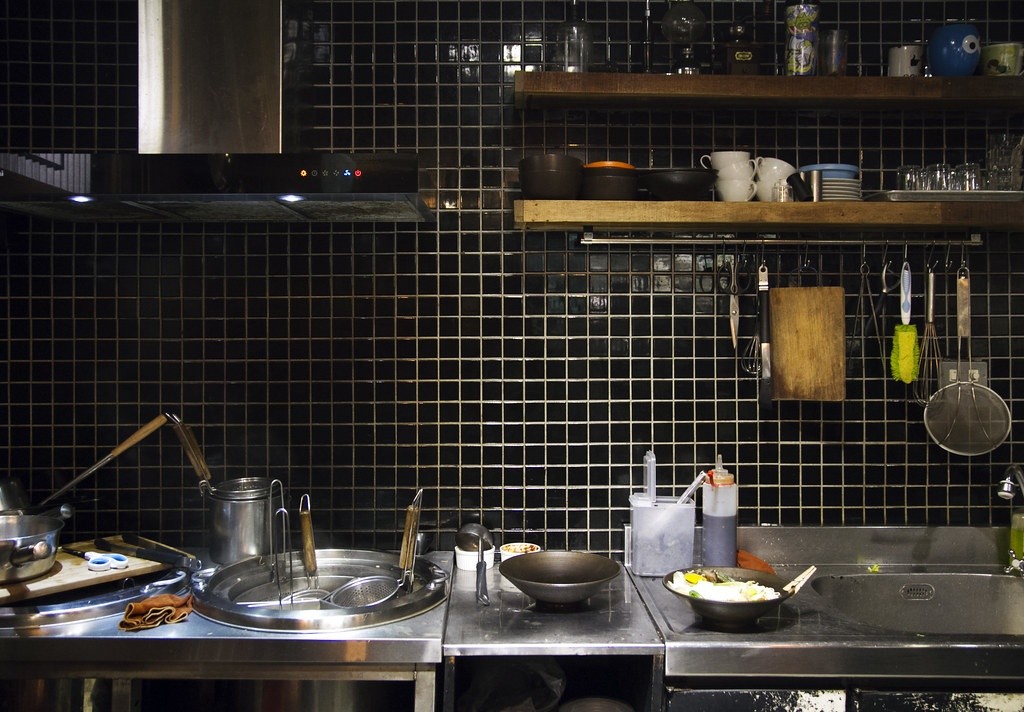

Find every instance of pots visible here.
[0,515,65,583]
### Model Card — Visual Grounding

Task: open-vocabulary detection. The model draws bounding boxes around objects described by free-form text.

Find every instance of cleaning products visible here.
[1009,507,1024,565]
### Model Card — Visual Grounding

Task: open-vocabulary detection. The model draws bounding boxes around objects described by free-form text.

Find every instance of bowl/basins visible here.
[800,164,858,178]
[519,154,638,200]
[639,168,719,201]
[662,566,794,621]
[454,544,495,569]
[499,551,622,604]
[207,489,286,564]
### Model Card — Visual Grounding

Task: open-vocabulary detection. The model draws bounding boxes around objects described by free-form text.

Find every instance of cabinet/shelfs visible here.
[513,70,1024,231]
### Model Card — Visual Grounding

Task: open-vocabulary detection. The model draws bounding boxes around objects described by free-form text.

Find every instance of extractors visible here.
[0,0,438,223]
[91,0,438,225]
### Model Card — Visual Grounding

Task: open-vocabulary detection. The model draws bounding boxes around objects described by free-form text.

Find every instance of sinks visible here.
[811,572,1023,637]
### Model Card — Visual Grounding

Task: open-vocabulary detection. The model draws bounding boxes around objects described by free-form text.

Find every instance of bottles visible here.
[785,5,821,77]
[1009,507,1024,566]
[701,455,739,568]
[556,5,595,72]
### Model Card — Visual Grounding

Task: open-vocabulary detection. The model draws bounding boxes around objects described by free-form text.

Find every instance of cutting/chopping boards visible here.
[769,267,846,401]
[0,534,196,604]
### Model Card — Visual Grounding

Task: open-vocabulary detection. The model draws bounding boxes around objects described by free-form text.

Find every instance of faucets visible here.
[997,464,1024,500]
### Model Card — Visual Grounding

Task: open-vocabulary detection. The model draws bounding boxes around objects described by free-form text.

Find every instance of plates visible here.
[822,179,862,201]
[500,543,540,555]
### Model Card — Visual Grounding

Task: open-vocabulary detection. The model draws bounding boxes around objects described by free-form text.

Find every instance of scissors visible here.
[717,261,752,349]
[59,542,130,572]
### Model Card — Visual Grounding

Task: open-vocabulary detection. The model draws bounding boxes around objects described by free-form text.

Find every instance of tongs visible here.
[94,533,190,568]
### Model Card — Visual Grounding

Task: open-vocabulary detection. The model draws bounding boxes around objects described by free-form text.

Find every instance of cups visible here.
[756,156,798,201]
[888,46,923,77]
[897,163,980,191]
[821,30,850,75]
[982,43,1024,76]
[701,151,758,202]
[988,135,1023,190]
